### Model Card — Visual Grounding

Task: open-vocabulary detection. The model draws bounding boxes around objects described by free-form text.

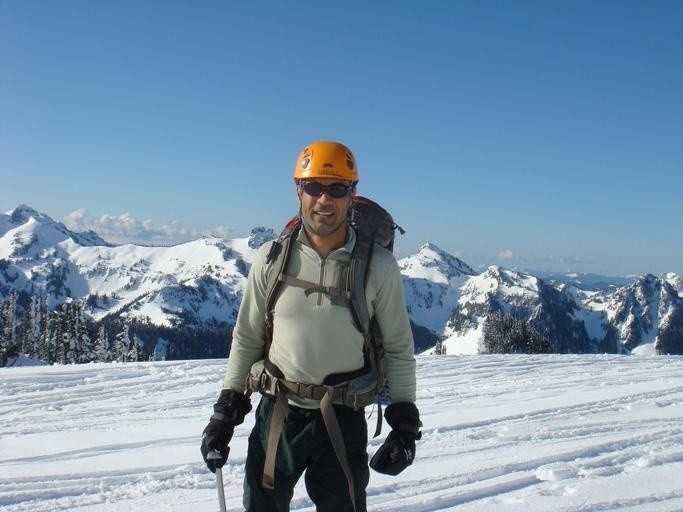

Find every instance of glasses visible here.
[298,180,359,198]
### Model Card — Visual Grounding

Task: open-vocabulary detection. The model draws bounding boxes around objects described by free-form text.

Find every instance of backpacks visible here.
[264,195,394,372]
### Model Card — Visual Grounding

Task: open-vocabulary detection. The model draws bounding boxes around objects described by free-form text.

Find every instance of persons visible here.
[196,140,423,511]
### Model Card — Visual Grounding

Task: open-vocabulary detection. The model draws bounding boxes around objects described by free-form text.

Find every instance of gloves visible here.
[369,401,422,475]
[201,389,252,474]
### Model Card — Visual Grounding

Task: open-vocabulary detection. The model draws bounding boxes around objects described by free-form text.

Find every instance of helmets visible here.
[294,141,359,182]
